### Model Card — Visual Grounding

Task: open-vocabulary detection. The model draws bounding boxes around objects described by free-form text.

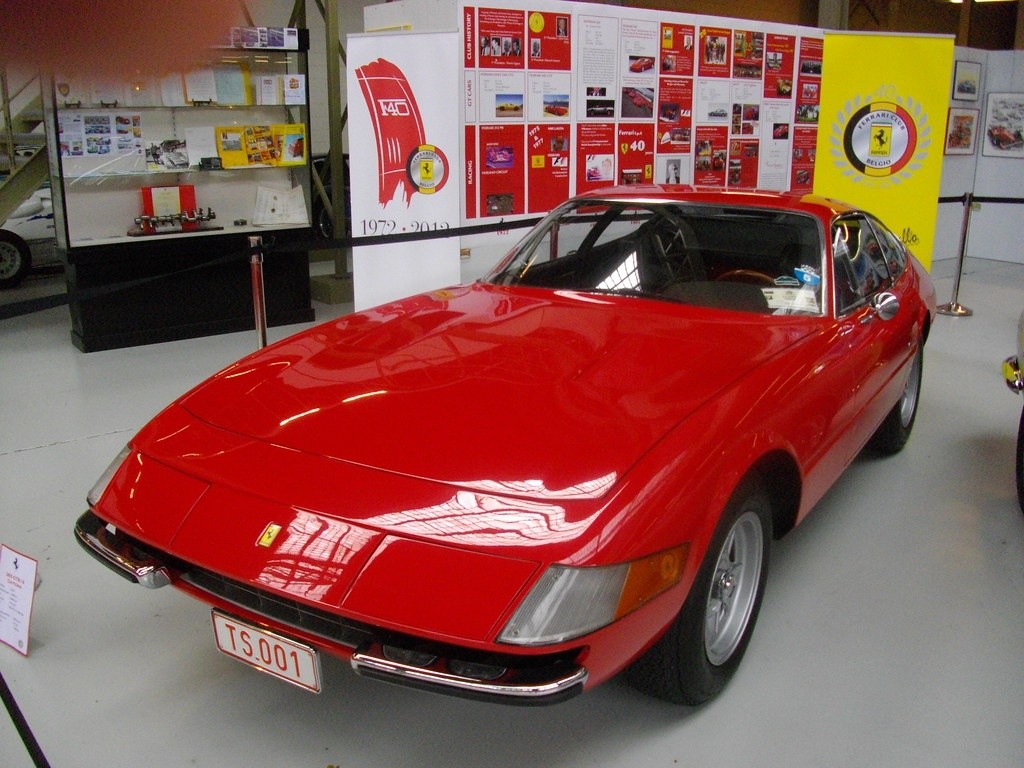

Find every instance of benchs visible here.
[652,214,878,300]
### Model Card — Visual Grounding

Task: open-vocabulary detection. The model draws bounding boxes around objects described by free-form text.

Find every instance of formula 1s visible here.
[989,125,1024,149]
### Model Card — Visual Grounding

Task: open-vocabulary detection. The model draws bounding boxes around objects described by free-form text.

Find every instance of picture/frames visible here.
[944,107,980,154]
[982,92,1024,158]
[952,60,982,101]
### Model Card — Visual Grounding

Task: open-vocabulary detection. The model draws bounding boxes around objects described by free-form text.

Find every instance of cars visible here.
[709,109,727,116]
[312,154,351,250]
[287,138,304,157]
[0,183,68,290]
[630,58,654,72]
[1001,312,1024,513]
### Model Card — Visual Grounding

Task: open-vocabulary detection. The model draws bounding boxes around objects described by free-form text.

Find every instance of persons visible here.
[666,163,680,184]
[480,37,521,56]
[705,40,726,64]
[558,19,567,36]
[531,42,540,57]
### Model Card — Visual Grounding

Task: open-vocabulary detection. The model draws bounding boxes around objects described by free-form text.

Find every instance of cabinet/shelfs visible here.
[41,49,316,353]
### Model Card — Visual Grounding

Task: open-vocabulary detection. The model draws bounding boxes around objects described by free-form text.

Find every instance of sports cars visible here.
[489,150,510,162]
[498,103,521,111]
[73,185,936,705]
[774,124,788,137]
[544,105,568,116]
